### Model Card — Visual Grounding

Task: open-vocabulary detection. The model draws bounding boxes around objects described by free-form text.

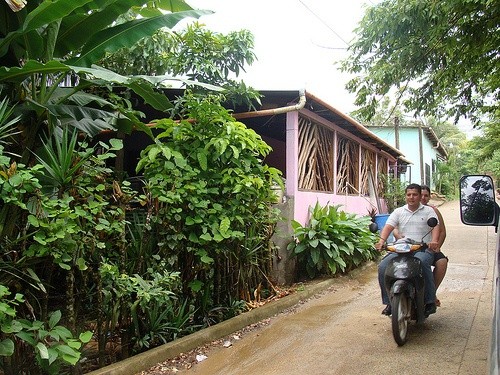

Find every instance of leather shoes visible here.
[381,305,391,315]
[426,303,437,314]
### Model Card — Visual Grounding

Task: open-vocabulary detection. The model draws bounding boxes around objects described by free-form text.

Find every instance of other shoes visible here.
[435,298,441,306]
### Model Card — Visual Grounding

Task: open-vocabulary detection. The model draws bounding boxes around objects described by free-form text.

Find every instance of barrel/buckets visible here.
[375,214,394,243]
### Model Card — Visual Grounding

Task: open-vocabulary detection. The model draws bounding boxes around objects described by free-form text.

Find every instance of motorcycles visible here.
[369,218,443,346]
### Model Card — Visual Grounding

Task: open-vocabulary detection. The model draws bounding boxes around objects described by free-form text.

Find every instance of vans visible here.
[458,174,500,375]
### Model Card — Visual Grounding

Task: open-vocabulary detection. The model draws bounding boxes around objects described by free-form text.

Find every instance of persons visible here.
[392,185,449,307]
[374,183,440,316]
